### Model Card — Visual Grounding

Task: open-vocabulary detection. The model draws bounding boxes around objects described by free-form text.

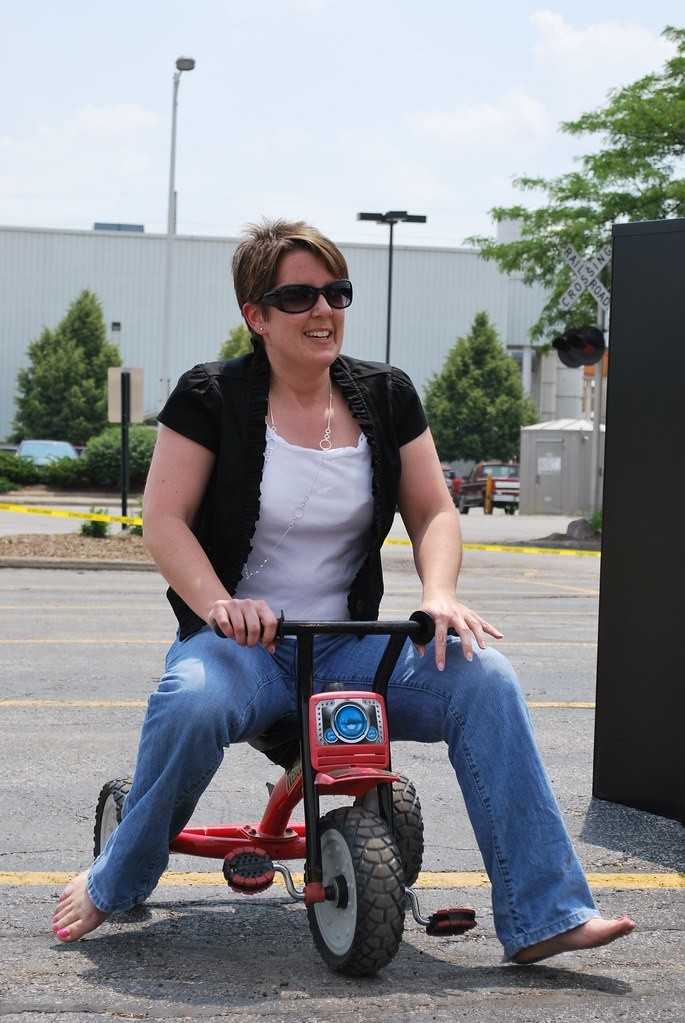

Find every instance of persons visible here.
[49,219,639,967]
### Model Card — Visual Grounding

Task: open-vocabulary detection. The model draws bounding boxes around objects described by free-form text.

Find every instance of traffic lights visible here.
[551,325,605,369]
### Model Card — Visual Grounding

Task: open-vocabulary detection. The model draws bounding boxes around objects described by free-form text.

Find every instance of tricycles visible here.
[90,607,480,979]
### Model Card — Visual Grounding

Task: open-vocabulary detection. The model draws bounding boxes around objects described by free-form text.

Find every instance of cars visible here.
[442,465,457,501]
[14,440,83,470]
[457,461,519,514]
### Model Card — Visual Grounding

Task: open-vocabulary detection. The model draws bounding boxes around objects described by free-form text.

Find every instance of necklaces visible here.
[245,378,332,584]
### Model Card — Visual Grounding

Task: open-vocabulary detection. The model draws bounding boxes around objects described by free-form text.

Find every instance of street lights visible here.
[154,55,199,389]
[355,207,429,367]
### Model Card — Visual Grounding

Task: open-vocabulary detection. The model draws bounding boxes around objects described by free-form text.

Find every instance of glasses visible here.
[261,279,353,314]
